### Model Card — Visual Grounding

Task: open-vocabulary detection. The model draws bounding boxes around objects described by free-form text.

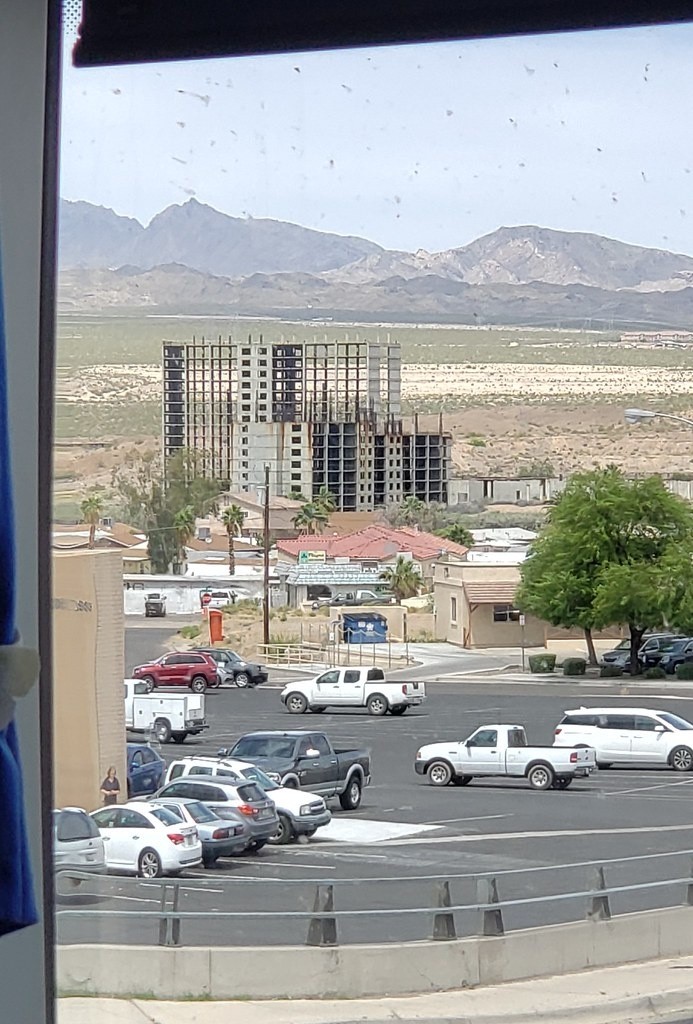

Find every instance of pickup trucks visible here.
[216,730,371,811]
[281,667,426,717]
[415,724,601,791]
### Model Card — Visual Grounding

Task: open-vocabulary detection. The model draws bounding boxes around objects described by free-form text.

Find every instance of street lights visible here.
[258,462,270,654]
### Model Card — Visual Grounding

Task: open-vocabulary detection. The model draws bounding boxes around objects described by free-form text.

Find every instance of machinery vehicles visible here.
[124,679,209,744]
[144,594,167,617]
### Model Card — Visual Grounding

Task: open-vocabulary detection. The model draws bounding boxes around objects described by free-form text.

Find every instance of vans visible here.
[553,706,693,771]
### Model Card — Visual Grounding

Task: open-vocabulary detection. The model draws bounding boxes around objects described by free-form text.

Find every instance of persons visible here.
[100,766,121,807]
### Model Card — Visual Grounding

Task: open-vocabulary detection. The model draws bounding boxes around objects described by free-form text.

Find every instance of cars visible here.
[150,797,247,868]
[88,802,204,879]
[643,638,693,674]
[128,744,166,798]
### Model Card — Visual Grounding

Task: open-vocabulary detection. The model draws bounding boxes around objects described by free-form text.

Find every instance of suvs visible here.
[128,774,279,857]
[207,591,234,610]
[164,754,332,843]
[52,807,106,878]
[188,647,269,689]
[603,633,686,673]
[132,651,218,693]
